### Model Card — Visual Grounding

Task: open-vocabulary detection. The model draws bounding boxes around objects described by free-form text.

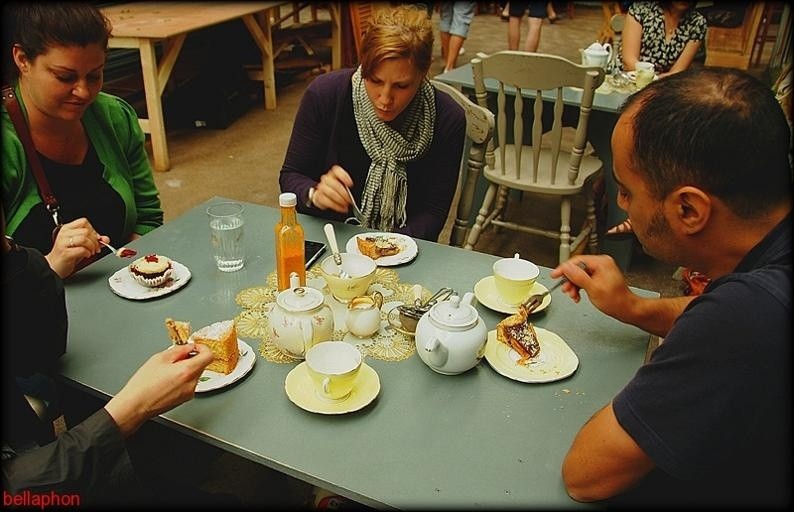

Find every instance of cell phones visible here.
[302,239,327,271]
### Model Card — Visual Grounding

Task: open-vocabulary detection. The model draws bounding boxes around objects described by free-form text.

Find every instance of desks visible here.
[97,0,282,171]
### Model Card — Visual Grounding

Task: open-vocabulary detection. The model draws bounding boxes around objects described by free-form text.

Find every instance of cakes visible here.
[191,319,239,375]
[128,253,174,288]
[355,236,407,259]
[496,307,540,360]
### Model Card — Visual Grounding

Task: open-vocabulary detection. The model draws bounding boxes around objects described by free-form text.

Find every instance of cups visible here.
[319,251,377,302]
[634,61,655,87]
[205,202,245,273]
[304,341,362,399]
[493,253,540,305]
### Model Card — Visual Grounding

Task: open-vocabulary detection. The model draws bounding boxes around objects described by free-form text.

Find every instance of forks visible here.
[523,263,589,311]
[345,183,368,222]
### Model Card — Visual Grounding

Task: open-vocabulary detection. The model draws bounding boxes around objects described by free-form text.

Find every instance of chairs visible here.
[705,0,764,71]
[465,52,605,265]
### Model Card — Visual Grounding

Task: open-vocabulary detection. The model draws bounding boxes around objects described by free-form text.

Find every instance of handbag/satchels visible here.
[2,87,97,275]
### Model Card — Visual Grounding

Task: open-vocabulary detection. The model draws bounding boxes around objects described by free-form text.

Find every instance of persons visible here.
[439,1,474,75]
[0,207,213,512]
[508,0,548,52]
[1,1,164,280]
[279,4,463,243]
[621,0,707,78]
[550,66,793,511]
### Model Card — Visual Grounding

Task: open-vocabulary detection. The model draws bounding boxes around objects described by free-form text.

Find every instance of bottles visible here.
[273,193,306,292]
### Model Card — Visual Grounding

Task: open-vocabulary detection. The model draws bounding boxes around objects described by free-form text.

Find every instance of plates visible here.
[346,233,418,266]
[108,257,192,300]
[284,360,381,415]
[473,276,552,314]
[486,326,579,383]
[192,339,257,394]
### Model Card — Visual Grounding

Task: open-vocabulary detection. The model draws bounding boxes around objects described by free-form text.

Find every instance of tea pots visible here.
[345,291,385,339]
[415,292,488,376]
[268,277,335,361]
[577,42,612,68]
